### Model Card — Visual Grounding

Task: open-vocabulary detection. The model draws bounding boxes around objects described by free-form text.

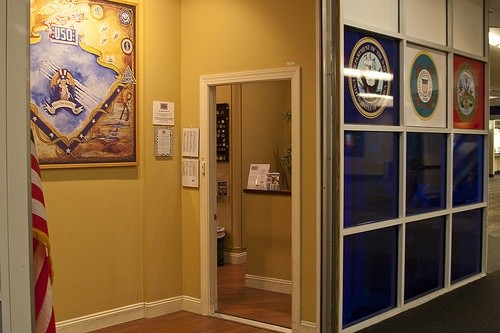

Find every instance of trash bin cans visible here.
[216,226,226,266]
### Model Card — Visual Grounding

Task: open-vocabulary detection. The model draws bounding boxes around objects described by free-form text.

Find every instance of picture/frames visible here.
[29,0,141,170]
